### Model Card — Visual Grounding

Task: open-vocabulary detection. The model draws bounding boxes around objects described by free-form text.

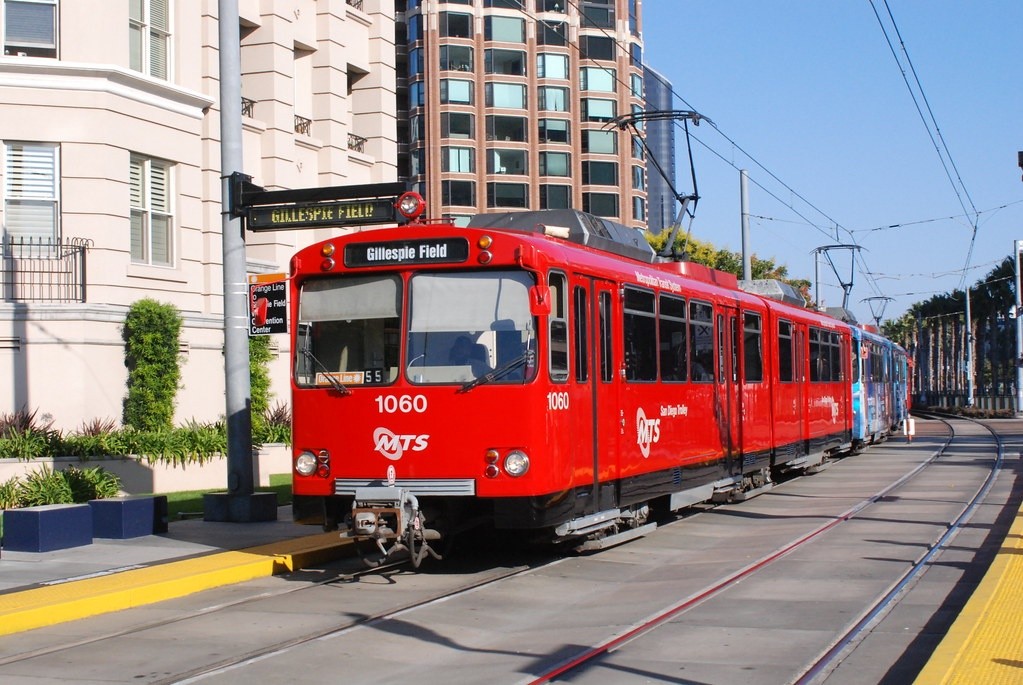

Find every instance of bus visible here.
[290,109,914,569]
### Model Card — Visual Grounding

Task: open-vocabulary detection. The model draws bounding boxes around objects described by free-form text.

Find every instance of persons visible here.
[448,336,492,377]
